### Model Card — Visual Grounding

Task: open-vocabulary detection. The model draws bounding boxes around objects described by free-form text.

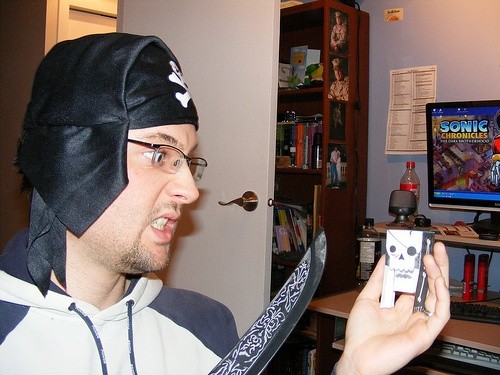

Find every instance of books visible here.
[275,123,322,170]
[274,206,309,253]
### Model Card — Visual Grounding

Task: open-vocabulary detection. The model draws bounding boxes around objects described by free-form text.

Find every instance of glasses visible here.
[127,139,207,182]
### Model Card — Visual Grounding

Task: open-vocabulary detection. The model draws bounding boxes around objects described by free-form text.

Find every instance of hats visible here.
[335,12,345,23]
[331,57,345,69]
[18,32,199,299]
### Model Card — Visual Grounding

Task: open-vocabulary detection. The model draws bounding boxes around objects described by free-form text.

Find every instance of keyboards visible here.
[428,341,500,369]
[449,302,500,324]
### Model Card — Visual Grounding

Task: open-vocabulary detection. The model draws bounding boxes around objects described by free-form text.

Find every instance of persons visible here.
[330,147,342,189]
[330,12,346,51]
[0,32,451,375]
[328,59,349,100]
[333,103,344,133]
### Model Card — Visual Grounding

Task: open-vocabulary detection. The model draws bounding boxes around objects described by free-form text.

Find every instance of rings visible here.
[424,310,432,316]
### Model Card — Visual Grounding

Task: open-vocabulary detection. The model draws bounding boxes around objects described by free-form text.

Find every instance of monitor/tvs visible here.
[425,100,500,208]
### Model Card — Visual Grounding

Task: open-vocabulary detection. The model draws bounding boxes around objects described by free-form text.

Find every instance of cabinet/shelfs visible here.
[268,0,369,375]
[306,221,500,375]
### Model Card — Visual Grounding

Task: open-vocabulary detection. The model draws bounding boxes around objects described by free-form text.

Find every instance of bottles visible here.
[400,161,420,219]
[312,132,322,169]
[356,218,382,293]
